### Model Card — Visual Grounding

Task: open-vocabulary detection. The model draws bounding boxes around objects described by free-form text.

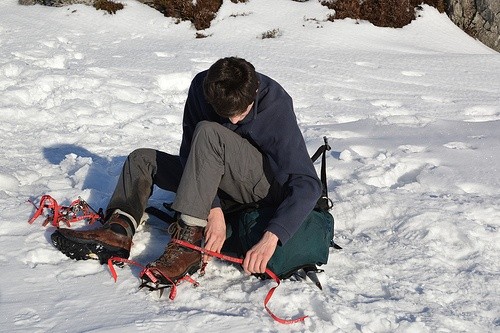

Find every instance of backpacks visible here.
[236,192,334,280]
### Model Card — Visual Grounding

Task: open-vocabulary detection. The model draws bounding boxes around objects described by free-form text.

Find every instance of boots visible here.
[142,218,204,284]
[51,213,133,265]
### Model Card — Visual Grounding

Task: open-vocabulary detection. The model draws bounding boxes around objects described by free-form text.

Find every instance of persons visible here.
[51,56,323,285]
